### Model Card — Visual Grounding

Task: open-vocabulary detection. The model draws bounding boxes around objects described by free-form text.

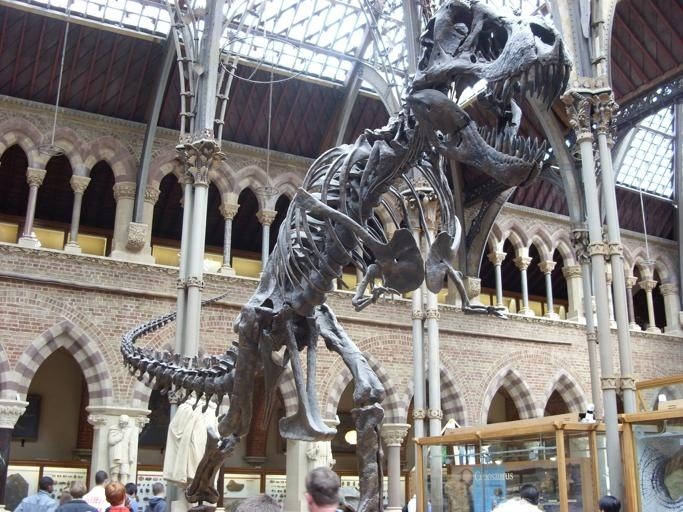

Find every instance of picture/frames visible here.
[138,389,170,450]
[11,394,41,442]
[332,411,358,453]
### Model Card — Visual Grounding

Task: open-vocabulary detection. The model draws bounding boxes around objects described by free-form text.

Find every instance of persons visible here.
[231,494,281,512]
[107,414,134,483]
[12,470,170,511]
[599,496,621,512]
[304,466,345,512]
[491,484,543,512]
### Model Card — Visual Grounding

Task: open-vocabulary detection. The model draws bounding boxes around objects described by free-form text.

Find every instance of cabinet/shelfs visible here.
[412,375,683,512]
